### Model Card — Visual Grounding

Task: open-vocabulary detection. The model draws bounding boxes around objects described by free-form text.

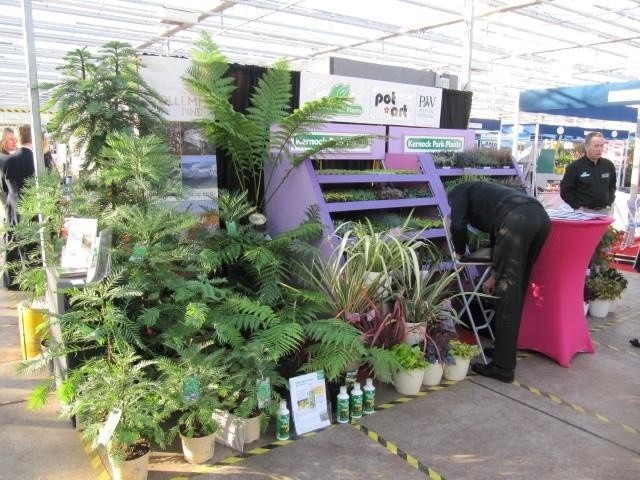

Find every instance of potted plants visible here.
[583,223,629,319]
[2,30,483,479]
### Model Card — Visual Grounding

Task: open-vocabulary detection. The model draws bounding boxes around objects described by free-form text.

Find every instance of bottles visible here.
[335,385,350,424]
[276,399,291,440]
[363,378,376,414]
[350,383,364,419]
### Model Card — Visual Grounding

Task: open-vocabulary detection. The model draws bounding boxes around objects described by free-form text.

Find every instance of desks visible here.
[514,215,616,369]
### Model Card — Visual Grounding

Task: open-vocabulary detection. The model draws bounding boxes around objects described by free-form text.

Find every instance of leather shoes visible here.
[472,361,514,383]
[484,347,516,368]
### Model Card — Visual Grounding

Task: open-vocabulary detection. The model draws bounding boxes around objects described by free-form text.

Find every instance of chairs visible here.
[439,213,496,367]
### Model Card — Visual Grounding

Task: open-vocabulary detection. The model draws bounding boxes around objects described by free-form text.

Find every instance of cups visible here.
[65,175,73,185]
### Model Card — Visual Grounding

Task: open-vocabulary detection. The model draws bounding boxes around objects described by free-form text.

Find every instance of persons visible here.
[629,248,640,348]
[0,124,57,292]
[448,181,550,384]
[560,132,617,268]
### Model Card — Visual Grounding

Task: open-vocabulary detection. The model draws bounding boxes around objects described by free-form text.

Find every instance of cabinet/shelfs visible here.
[425,150,535,282]
